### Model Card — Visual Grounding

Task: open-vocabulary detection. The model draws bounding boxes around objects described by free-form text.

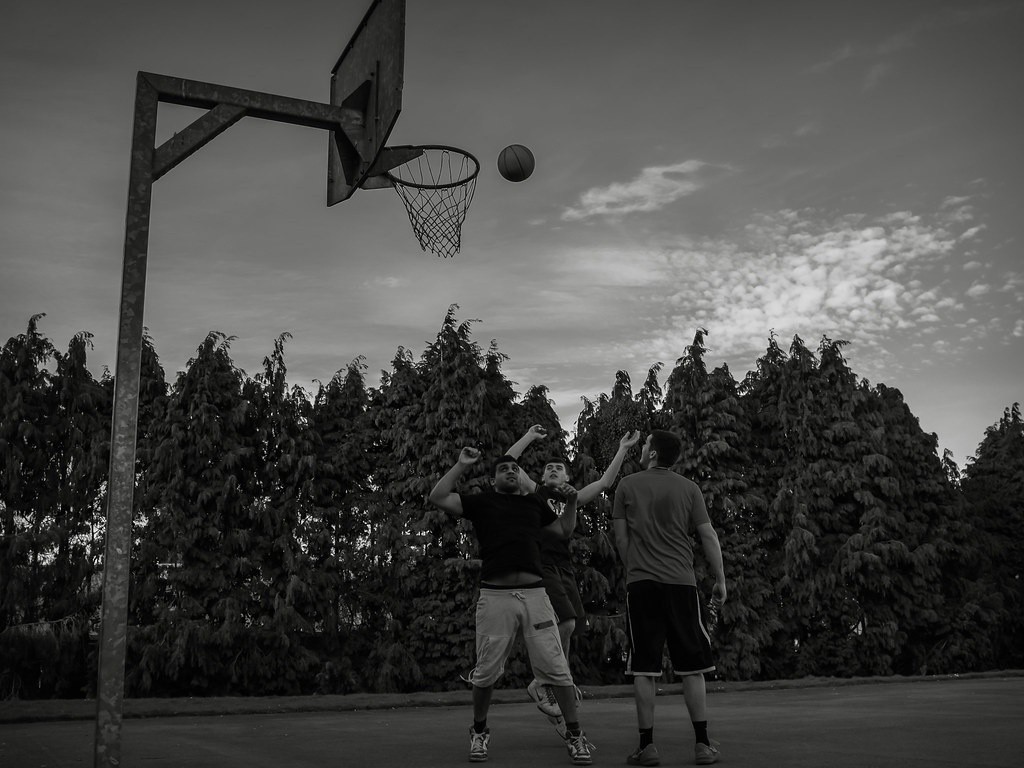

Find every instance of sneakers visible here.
[526,679,563,717]
[693,742,722,764]
[626,742,661,767]
[563,729,597,764]
[547,715,567,740]
[468,724,492,762]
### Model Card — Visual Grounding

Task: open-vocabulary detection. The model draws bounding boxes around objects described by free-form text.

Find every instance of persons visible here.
[610,430,728,768]
[501,423,642,740]
[429,446,596,766]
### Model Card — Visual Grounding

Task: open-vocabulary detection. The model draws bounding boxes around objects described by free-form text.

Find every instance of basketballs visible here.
[497,145,535,182]
